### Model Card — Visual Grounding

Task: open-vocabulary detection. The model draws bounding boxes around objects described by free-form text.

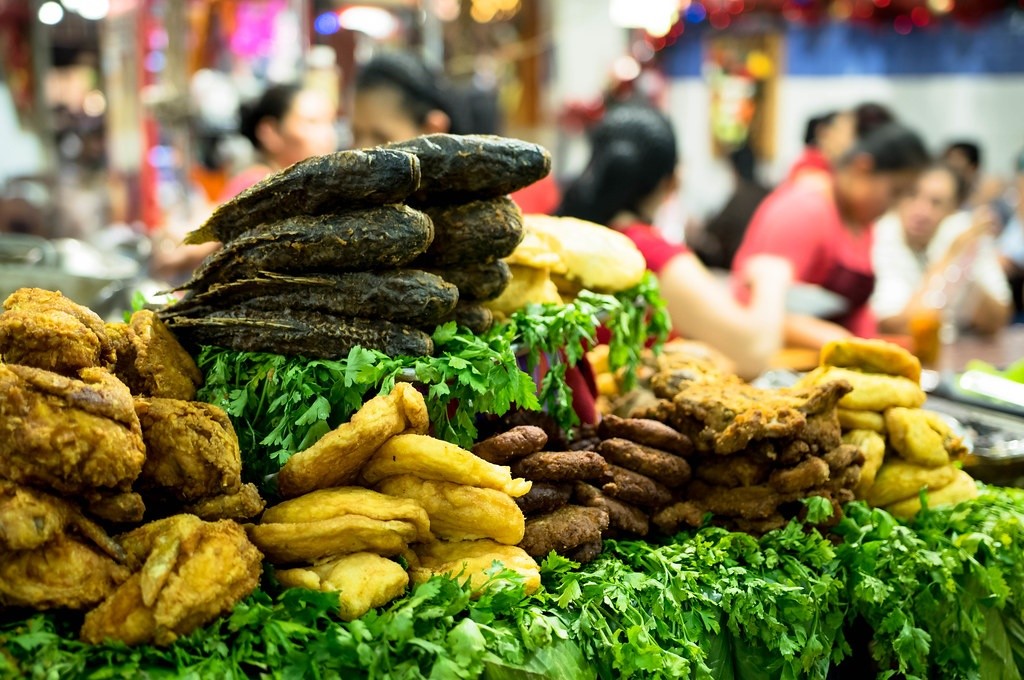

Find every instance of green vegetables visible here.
[191,276,664,479]
[0,499,1024,679]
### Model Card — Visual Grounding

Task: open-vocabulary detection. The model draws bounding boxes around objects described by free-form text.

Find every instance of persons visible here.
[182,50,1024,381]
